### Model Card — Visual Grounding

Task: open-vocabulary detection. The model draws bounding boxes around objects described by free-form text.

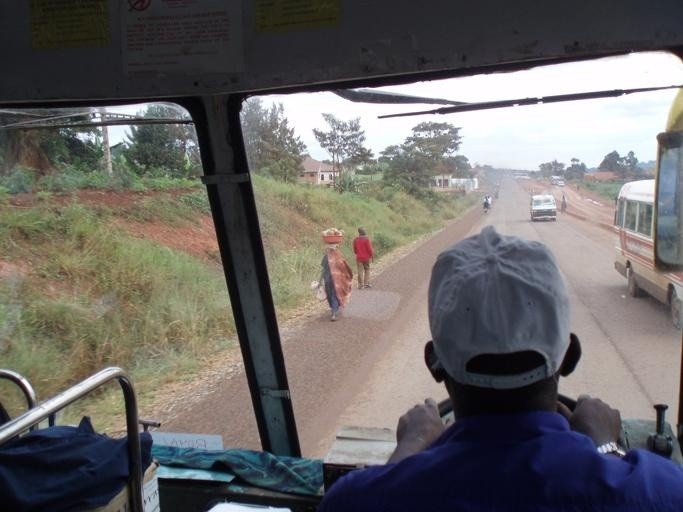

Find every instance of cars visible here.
[529,194,557,222]
[550,176,565,186]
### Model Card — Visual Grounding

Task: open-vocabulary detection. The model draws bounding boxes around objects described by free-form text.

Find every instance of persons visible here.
[352,226,374,290]
[317,225,683,512]
[484,195,491,212]
[561,196,566,213]
[315,242,353,322]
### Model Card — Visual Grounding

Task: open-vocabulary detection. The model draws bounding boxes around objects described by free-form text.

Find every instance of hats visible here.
[427,227,571,390]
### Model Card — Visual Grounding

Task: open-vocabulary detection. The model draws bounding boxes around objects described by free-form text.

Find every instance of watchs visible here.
[596,442,627,457]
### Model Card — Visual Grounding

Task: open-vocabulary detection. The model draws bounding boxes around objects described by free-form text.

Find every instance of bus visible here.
[613,179,683,330]
